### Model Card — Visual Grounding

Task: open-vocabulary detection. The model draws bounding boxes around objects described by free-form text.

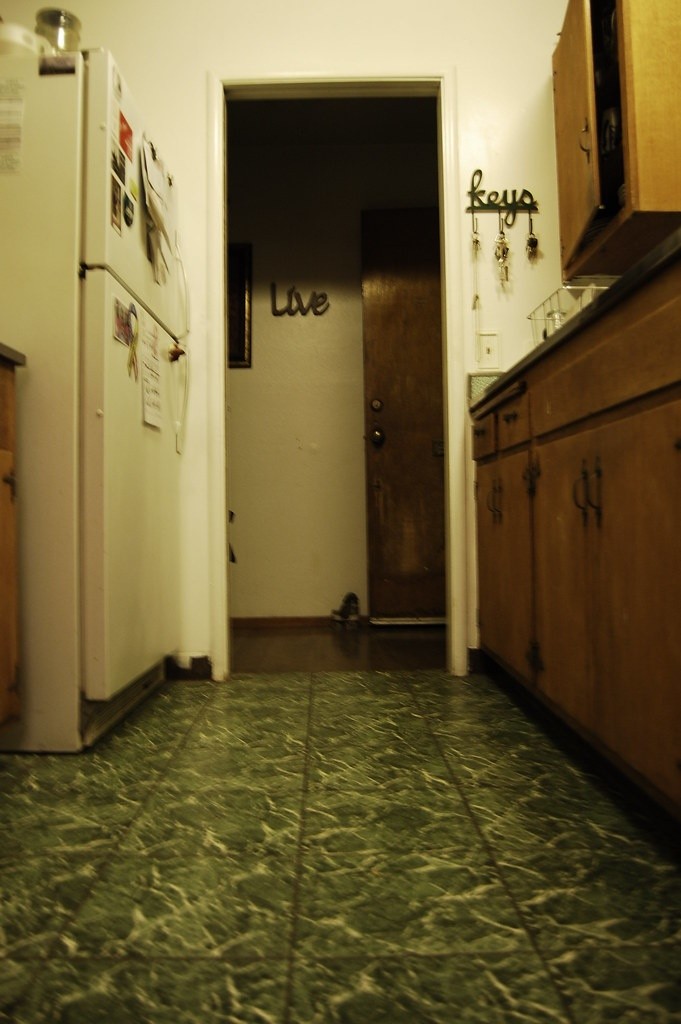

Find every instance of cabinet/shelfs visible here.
[479,228,681,861]
[549,0,681,284]
[0,360,27,735]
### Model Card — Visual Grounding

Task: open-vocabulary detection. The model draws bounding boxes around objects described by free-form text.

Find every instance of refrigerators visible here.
[0,49,182,751]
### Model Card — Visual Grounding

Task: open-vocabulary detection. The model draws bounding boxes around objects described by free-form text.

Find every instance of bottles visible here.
[34,6,78,52]
